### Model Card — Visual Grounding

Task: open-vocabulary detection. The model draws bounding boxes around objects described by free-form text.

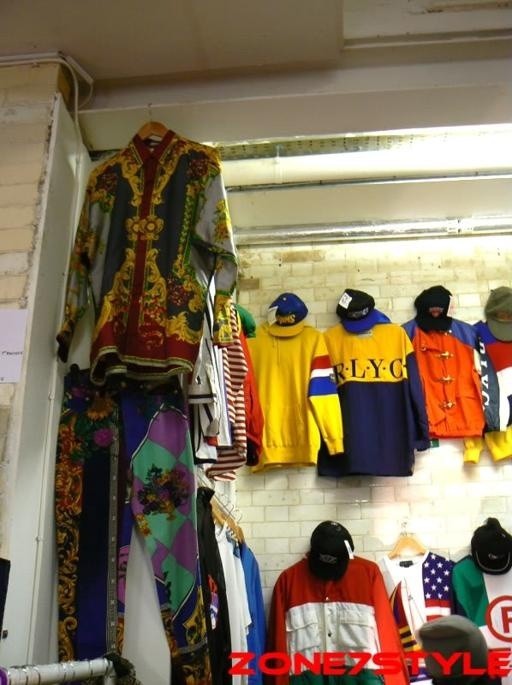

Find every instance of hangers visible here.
[137,102,169,145]
[387,515,426,560]
[195,464,247,544]
[0,656,116,685]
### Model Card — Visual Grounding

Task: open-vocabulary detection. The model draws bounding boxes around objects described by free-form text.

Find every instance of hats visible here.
[235,304,256,338]
[472,517,512,574]
[414,285,456,330]
[308,520,354,581]
[266,293,308,338]
[485,286,512,341]
[336,288,391,332]
